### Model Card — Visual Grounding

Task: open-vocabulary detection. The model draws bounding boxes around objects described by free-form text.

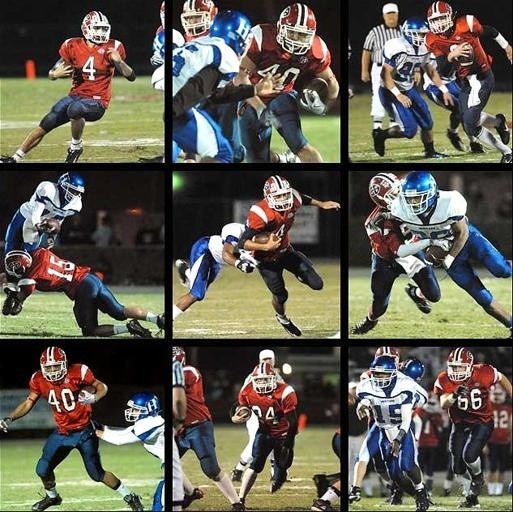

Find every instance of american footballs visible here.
[424,236,453,265]
[459,44,478,69]
[78,388,99,403]
[252,234,281,252]
[234,408,253,423]
[304,79,326,107]
[39,219,59,238]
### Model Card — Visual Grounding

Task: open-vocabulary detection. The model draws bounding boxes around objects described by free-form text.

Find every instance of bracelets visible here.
[444,254,455,268]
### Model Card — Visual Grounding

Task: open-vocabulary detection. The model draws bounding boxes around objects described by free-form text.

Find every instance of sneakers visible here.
[311,475,334,512]
[440,481,481,509]
[501,149,513,163]
[126,312,166,339]
[422,148,449,158]
[231,466,243,481]
[230,498,246,512]
[65,147,83,163]
[495,114,510,145]
[351,315,378,334]
[138,156,163,163]
[270,466,287,493]
[276,314,301,336]
[405,284,431,314]
[176,259,190,287]
[447,128,485,153]
[347,477,433,511]
[124,492,144,512]
[2,291,22,315]
[0,153,16,163]
[373,129,385,156]
[284,148,301,163]
[32,487,62,512]
[373,122,400,130]
[182,488,203,508]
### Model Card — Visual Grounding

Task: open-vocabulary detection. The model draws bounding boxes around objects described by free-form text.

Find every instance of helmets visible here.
[180,0,218,37]
[427,1,457,34]
[210,10,252,55]
[40,346,68,382]
[402,171,438,216]
[402,16,431,47]
[369,173,402,209]
[276,2,317,55]
[172,346,185,365]
[124,392,161,423]
[263,175,294,212]
[4,250,33,278]
[81,11,111,44]
[446,348,474,382]
[490,388,506,405]
[369,346,425,392]
[250,363,278,394]
[58,171,85,203]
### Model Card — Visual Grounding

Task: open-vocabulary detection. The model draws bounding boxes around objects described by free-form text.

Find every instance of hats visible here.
[382,3,398,13]
[259,349,275,359]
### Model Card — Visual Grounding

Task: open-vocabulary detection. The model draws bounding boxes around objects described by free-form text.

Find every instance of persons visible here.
[172,346,341,512]
[347,345,513,512]
[172,222,258,321]
[138,0,341,163]
[2,171,85,315]
[350,172,441,335]
[2,247,165,339]
[348,0,513,163]
[237,174,341,337]
[0,345,144,512]
[391,170,512,339]
[0,10,136,163]
[95,392,165,512]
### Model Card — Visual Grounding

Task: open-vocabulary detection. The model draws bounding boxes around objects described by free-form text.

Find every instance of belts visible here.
[377,62,382,66]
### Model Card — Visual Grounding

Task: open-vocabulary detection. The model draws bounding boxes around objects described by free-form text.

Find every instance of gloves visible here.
[0,417,14,432]
[151,55,164,69]
[36,218,56,250]
[453,386,468,398]
[238,260,256,274]
[78,390,95,407]
[430,239,455,270]
[299,89,326,116]
[258,107,282,130]
